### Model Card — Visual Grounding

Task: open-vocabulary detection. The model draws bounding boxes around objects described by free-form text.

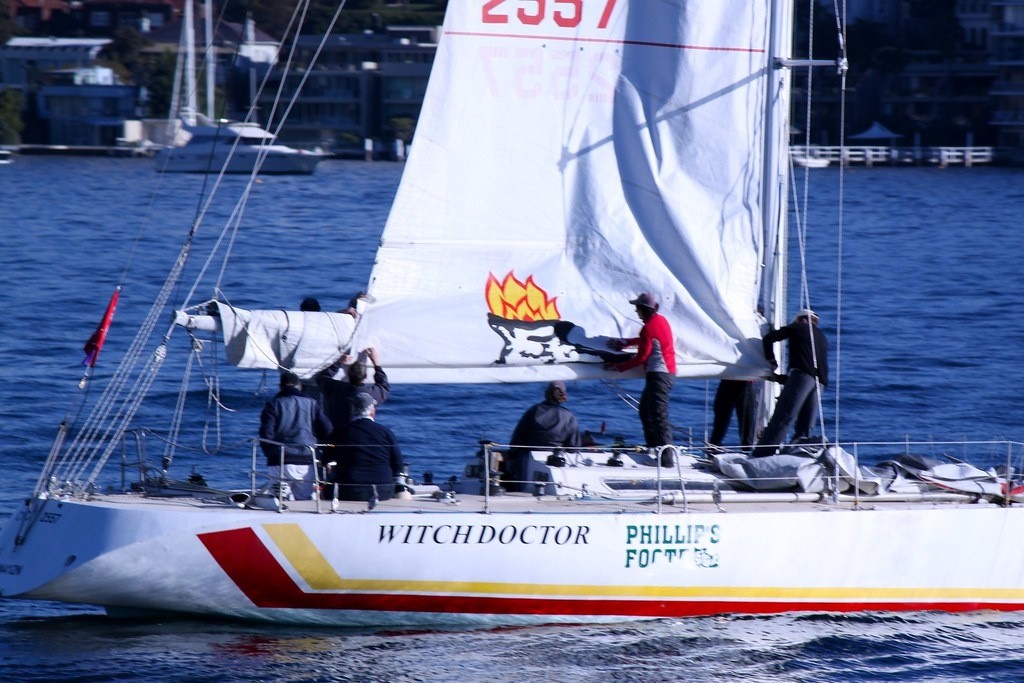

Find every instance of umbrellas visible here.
[790,125,802,133]
[848,121,903,139]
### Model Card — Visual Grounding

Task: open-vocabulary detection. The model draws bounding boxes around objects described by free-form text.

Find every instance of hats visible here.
[629,292,657,309]
[793,309,819,325]
[280,372,300,384]
[547,380,565,396]
[349,361,367,379]
[354,393,379,409]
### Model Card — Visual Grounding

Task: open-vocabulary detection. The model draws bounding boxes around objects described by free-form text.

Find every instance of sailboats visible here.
[0,0,1024,635]
[115,0,338,177]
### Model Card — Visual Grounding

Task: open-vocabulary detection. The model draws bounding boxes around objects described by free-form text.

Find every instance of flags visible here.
[84,292,121,356]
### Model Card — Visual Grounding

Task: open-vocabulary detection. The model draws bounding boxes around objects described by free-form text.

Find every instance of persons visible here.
[300,292,368,393]
[753,310,827,458]
[708,378,758,454]
[315,347,390,429]
[261,373,334,500]
[317,392,411,502]
[600,293,675,467]
[507,380,598,491]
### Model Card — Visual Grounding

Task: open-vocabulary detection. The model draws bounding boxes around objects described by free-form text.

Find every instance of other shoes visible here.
[644,458,662,467]
